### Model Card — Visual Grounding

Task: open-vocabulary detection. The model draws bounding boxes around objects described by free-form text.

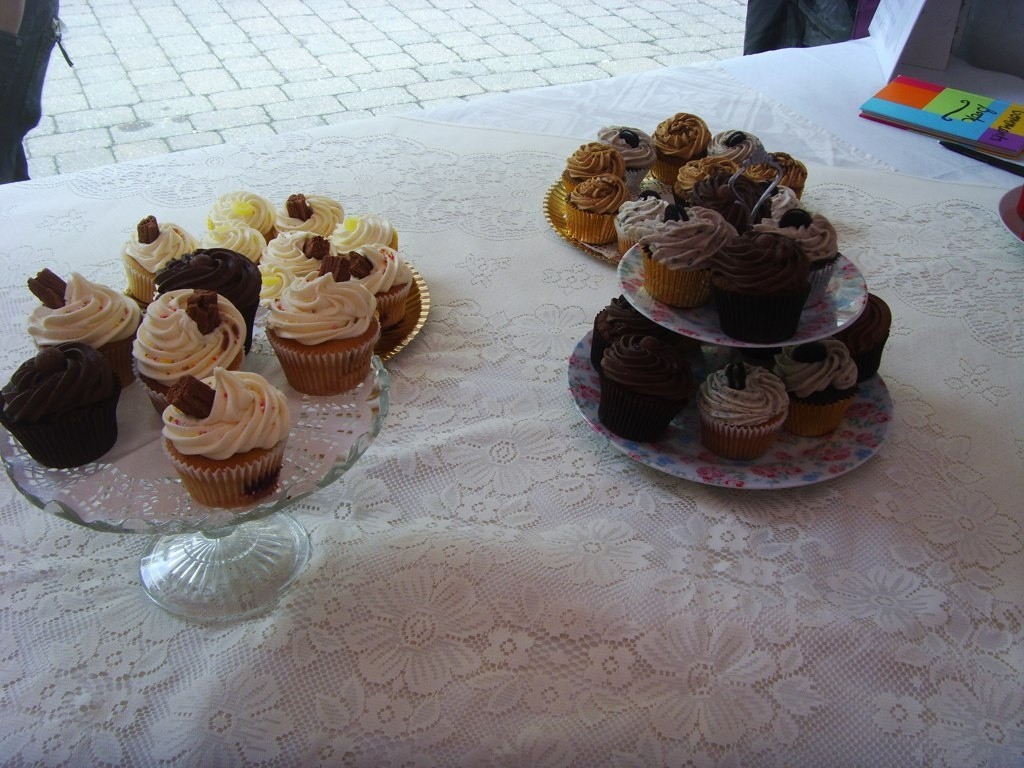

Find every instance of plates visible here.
[542,177,618,267]
[566,329,894,489]
[377,258,432,363]
[617,241,869,348]
[997,183,1024,243]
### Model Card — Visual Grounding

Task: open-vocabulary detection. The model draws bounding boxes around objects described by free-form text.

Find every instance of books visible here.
[857,73,1024,161]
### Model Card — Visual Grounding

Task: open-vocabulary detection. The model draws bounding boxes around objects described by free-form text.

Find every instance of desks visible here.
[0,37,1024,768]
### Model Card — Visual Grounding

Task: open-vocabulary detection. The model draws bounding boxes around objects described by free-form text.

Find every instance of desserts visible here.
[591,293,891,460]
[0,190,413,507]
[641,175,840,343]
[562,113,807,256]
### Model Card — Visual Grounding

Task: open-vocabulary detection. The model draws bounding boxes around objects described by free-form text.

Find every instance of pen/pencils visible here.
[938,140,1024,177]
